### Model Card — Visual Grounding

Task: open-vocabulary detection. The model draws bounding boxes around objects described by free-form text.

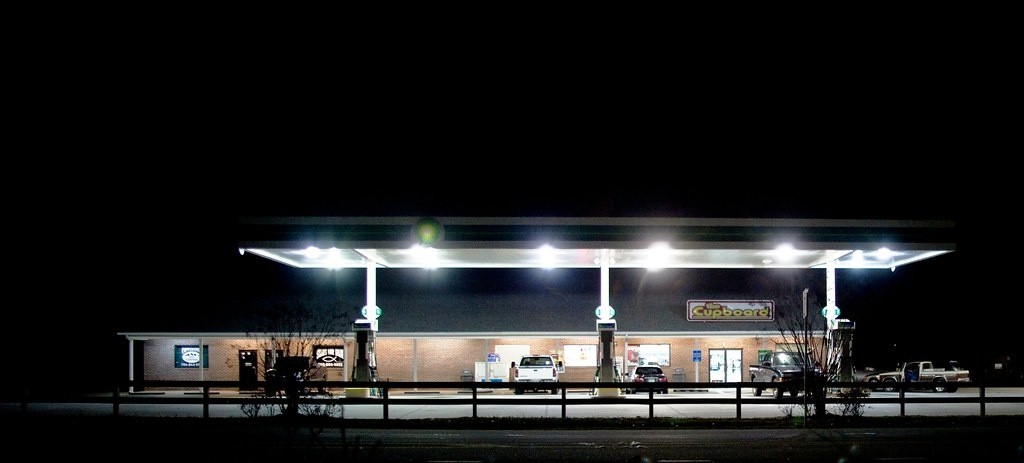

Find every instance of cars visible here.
[863,373,880,383]
[264,355,330,396]
[625,365,669,394]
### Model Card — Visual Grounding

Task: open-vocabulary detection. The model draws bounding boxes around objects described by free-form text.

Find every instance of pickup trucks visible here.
[749,352,829,398]
[879,361,970,393]
[515,353,566,394]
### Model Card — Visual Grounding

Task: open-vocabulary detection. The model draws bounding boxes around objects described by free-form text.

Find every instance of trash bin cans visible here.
[673,368,686,382]
[460,370,474,381]
